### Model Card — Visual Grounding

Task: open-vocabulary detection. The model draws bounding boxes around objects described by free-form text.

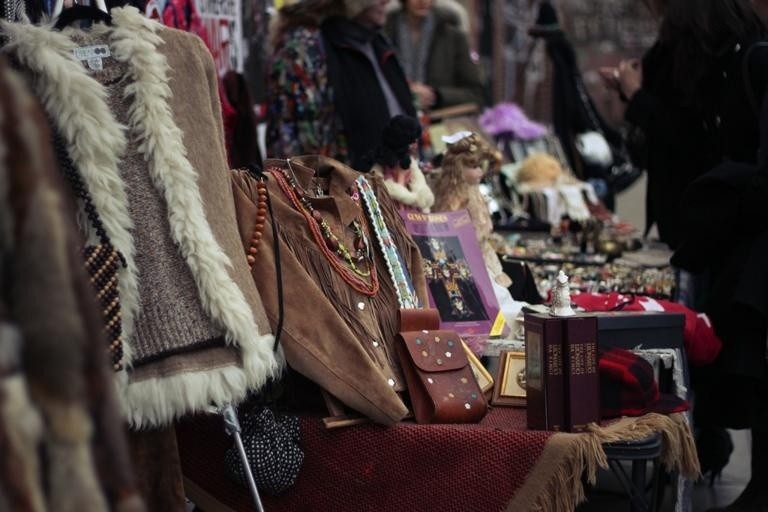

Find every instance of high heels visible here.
[695,455,729,486]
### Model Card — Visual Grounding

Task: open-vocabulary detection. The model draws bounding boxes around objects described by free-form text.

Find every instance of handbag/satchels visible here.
[394,307,488,426]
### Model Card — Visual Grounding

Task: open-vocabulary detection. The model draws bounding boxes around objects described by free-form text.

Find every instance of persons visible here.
[369,112,435,212]
[243,1,496,172]
[431,125,514,289]
[613,0,768,512]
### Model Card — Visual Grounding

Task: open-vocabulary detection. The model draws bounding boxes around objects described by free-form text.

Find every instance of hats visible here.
[478,102,551,139]
[598,344,691,416]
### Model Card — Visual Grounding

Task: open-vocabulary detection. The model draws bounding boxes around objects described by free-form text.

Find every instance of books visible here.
[395,204,511,342]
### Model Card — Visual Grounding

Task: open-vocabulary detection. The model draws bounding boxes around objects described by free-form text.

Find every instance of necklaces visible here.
[244,164,423,308]
